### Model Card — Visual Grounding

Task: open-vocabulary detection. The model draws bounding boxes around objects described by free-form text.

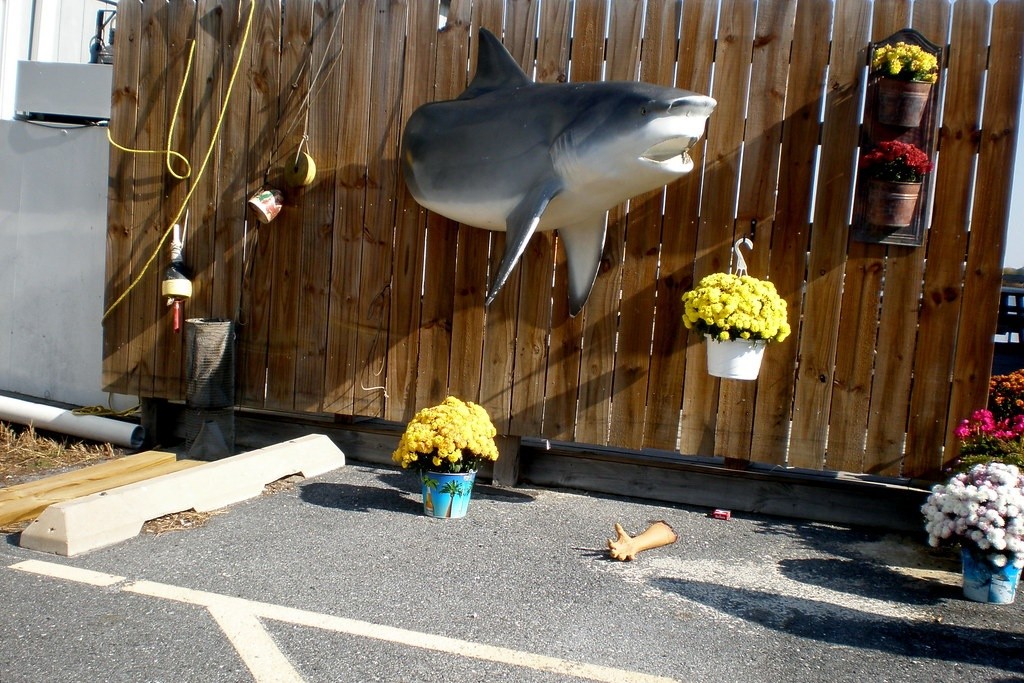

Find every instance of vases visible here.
[877,77,933,127]
[867,174,922,228]
[959,545,1022,605]
[419,468,478,519]
[701,333,769,381]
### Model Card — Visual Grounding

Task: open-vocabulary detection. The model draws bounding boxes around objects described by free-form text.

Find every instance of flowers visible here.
[918,462,1024,567]
[869,40,939,84]
[390,395,500,474]
[680,274,792,348]
[857,140,934,182]
[953,369,1023,470]
